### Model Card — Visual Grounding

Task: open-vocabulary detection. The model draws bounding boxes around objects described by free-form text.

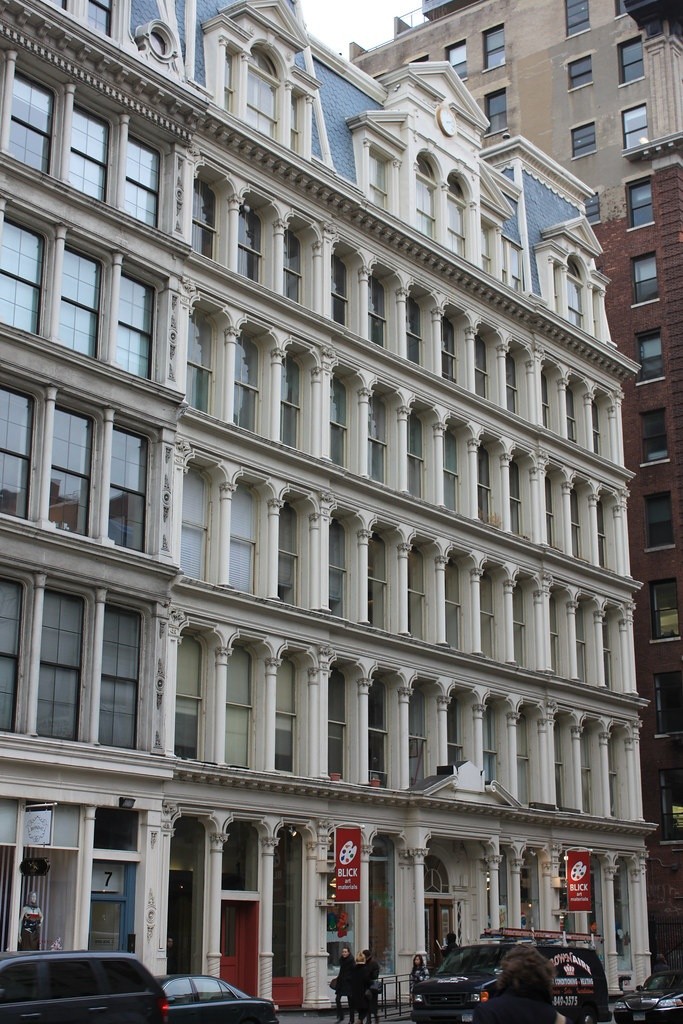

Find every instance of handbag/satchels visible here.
[330,977,338,990]
[369,980,383,994]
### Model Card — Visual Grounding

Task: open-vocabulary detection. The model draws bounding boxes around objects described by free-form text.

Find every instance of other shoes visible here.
[347,1021,354,1024]
[335,1017,344,1023]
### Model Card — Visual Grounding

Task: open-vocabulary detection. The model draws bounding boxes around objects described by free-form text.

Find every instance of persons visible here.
[440,933,459,956]
[18,890,44,951]
[334,947,380,1024]
[471,944,574,1024]
[409,954,430,1003]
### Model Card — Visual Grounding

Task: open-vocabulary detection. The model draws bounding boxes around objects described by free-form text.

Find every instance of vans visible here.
[0,950,168,1023]
[410,943,614,1024]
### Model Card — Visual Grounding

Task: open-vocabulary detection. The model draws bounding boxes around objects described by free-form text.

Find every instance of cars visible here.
[613,969,682,1024]
[154,972,284,1024]
